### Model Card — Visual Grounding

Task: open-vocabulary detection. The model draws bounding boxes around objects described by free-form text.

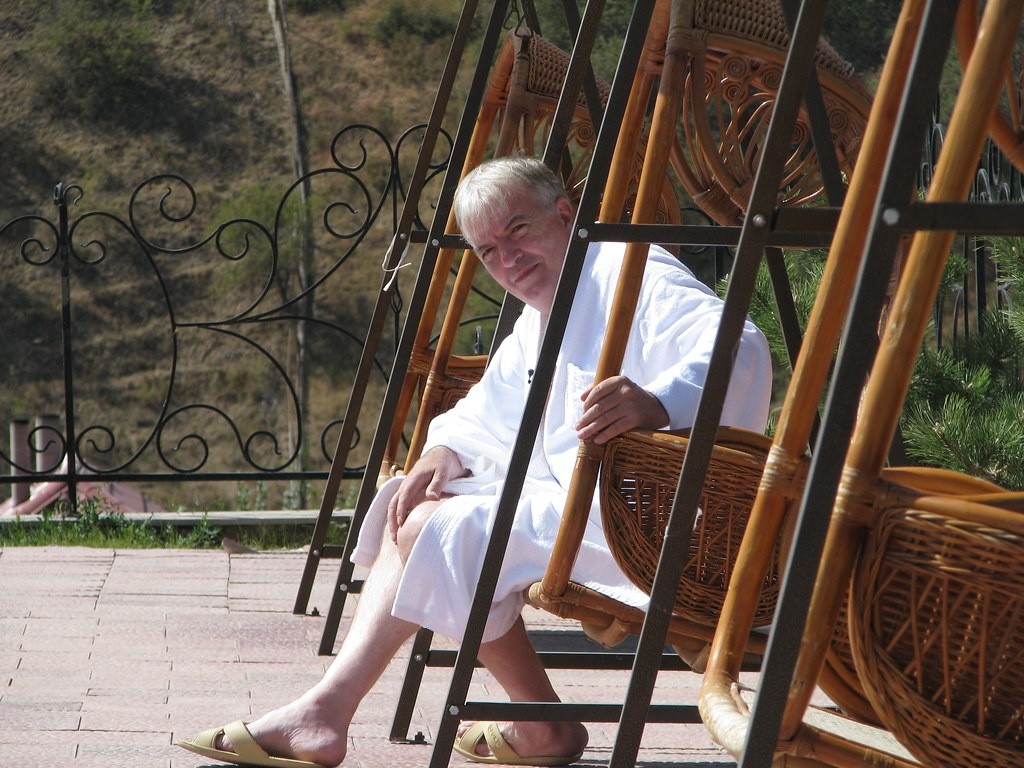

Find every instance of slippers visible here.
[176,720,324,768]
[453,721,583,765]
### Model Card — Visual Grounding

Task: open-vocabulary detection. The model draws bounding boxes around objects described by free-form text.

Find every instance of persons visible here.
[170,155,772,768]
